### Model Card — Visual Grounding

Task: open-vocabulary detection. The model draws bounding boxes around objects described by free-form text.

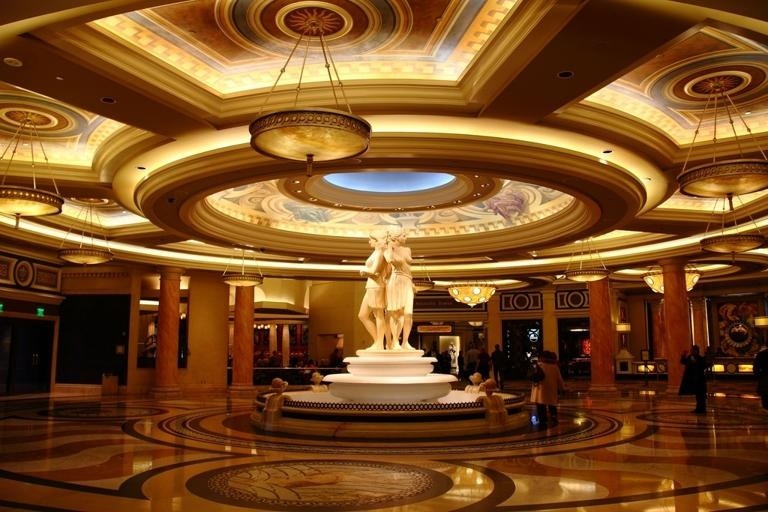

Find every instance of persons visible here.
[489,344,505,390]
[751,337,768,411]
[356,229,385,351]
[382,226,416,349]
[422,342,491,381]
[678,345,707,414]
[528,350,566,427]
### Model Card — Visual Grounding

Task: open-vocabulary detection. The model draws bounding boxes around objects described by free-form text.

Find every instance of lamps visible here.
[564,239,610,282]
[57,199,114,265]
[676,83,768,211]
[754,316,768,327]
[220,249,264,287]
[447,280,496,308]
[615,323,630,331]
[700,196,765,263]
[640,263,703,295]
[0,113,64,229]
[248,16,372,176]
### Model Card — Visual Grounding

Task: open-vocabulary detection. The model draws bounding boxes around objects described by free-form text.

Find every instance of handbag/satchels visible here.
[527,366,545,383]
